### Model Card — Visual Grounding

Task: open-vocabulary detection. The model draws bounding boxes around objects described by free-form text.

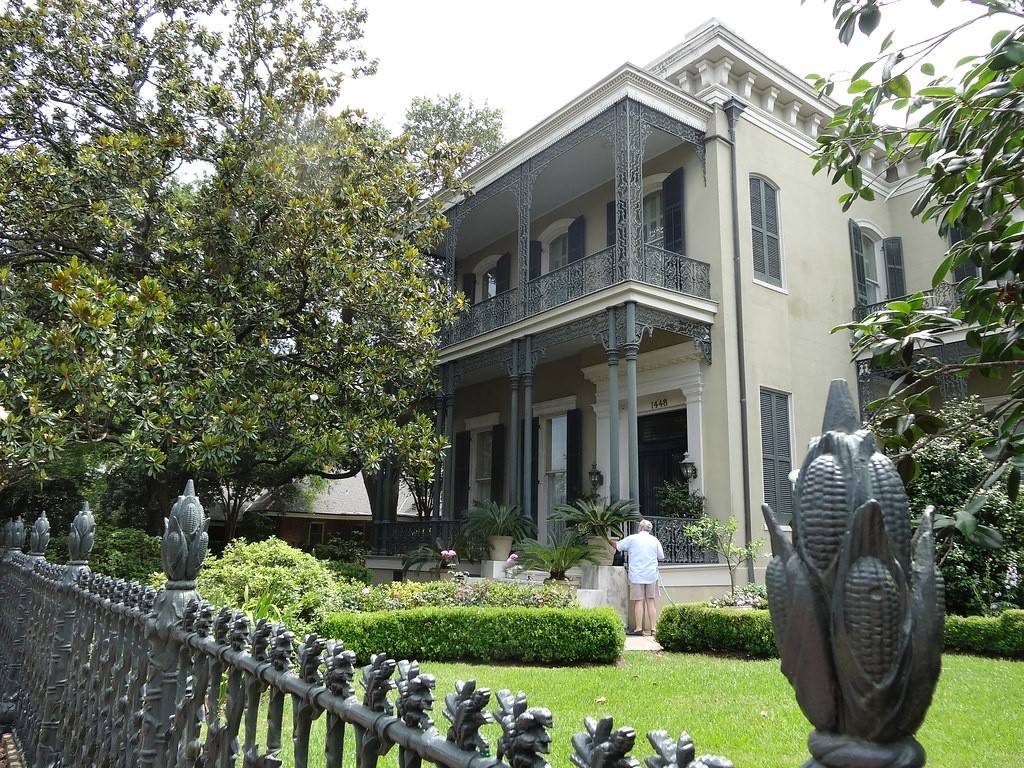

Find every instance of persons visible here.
[609,520,664,636]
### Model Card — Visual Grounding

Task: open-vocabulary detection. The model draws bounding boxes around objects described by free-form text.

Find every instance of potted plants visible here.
[401,537,471,581]
[546,496,642,566]
[460,498,538,560]
[510,529,607,596]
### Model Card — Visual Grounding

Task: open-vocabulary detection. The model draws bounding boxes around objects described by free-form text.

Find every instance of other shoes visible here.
[626,629,643,636]
[651,630,656,635]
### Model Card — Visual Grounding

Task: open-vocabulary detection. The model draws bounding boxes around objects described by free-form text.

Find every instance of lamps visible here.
[679,451,697,479]
[588,460,604,487]
